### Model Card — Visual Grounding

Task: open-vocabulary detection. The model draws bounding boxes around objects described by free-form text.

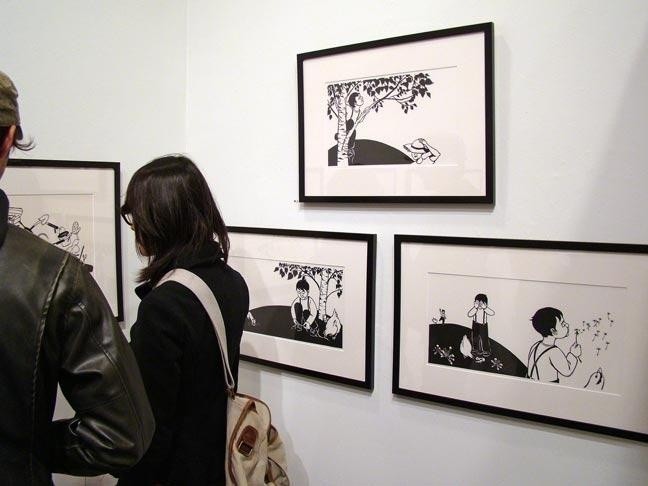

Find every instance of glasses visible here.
[121,202,136,228]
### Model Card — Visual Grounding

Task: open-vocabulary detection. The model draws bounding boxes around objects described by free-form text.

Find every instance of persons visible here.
[115,151,253,485]
[0,69,158,486]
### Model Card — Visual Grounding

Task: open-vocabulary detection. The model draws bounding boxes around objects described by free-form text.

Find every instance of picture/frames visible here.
[297,19,497,208]
[219,225,376,391]
[3,157,123,327]
[389,232,648,444]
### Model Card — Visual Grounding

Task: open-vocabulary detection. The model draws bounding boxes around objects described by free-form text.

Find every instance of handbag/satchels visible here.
[223,388,291,486]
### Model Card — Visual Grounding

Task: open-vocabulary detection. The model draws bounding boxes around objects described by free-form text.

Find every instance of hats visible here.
[403,139,428,154]
[0,71,24,140]
